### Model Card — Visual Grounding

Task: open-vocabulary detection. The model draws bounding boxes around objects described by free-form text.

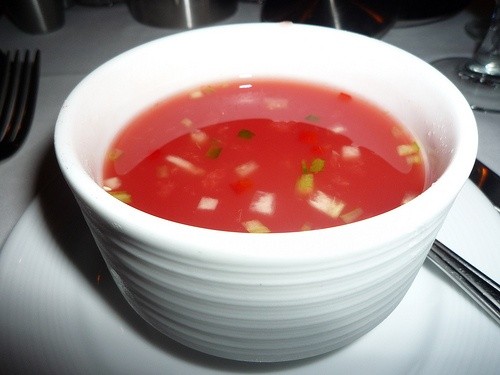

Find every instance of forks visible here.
[0,47,42,163]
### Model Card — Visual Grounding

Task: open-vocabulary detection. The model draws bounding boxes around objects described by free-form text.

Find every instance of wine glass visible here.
[425,0,500,120]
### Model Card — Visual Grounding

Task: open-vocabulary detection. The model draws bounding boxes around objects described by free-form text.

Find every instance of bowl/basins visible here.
[51,21,480,364]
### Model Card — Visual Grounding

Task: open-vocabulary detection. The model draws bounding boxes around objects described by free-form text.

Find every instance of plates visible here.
[1,167,500,375]
